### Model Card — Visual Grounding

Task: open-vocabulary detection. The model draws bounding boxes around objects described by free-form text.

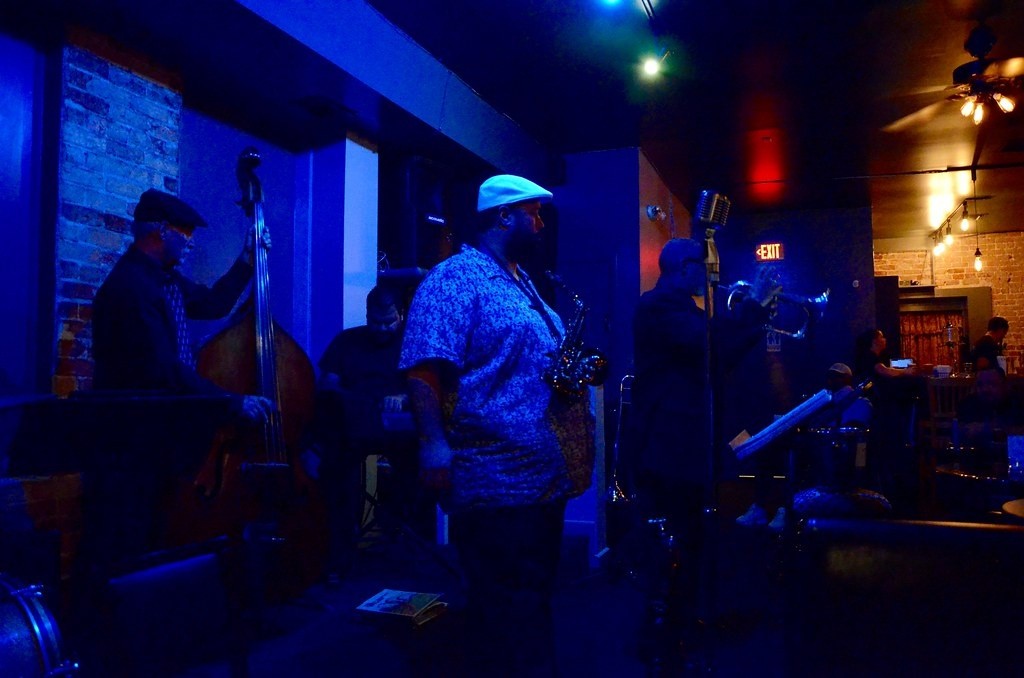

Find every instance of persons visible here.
[736,317,1024,527]
[68,190,272,678]
[632,237,772,643]
[395,175,595,678]
[317,284,449,589]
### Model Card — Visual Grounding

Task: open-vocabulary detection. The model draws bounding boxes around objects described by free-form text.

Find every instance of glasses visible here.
[830,373,844,378]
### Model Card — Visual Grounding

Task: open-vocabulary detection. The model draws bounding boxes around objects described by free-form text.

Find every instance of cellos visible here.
[161,148,332,595]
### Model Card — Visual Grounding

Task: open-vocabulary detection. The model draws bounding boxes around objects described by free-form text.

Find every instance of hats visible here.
[477,175,552,212]
[133,188,208,227]
[827,363,853,376]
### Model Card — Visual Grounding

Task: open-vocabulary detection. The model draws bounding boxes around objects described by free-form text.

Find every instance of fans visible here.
[880,26,1024,135]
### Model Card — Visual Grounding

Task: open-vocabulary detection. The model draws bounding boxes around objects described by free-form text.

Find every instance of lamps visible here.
[960,82,1018,126]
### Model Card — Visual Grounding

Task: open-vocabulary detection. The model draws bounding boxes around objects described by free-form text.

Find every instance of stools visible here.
[918,374,980,516]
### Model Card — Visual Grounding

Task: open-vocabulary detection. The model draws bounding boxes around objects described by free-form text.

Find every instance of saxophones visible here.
[540,261,609,401]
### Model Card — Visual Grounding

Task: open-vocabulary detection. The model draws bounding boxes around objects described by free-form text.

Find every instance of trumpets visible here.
[717,280,831,339]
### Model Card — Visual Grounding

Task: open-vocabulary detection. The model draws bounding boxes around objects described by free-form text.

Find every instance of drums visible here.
[0,580,71,678]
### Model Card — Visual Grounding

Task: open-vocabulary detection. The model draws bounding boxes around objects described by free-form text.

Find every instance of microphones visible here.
[694,190,731,287]
